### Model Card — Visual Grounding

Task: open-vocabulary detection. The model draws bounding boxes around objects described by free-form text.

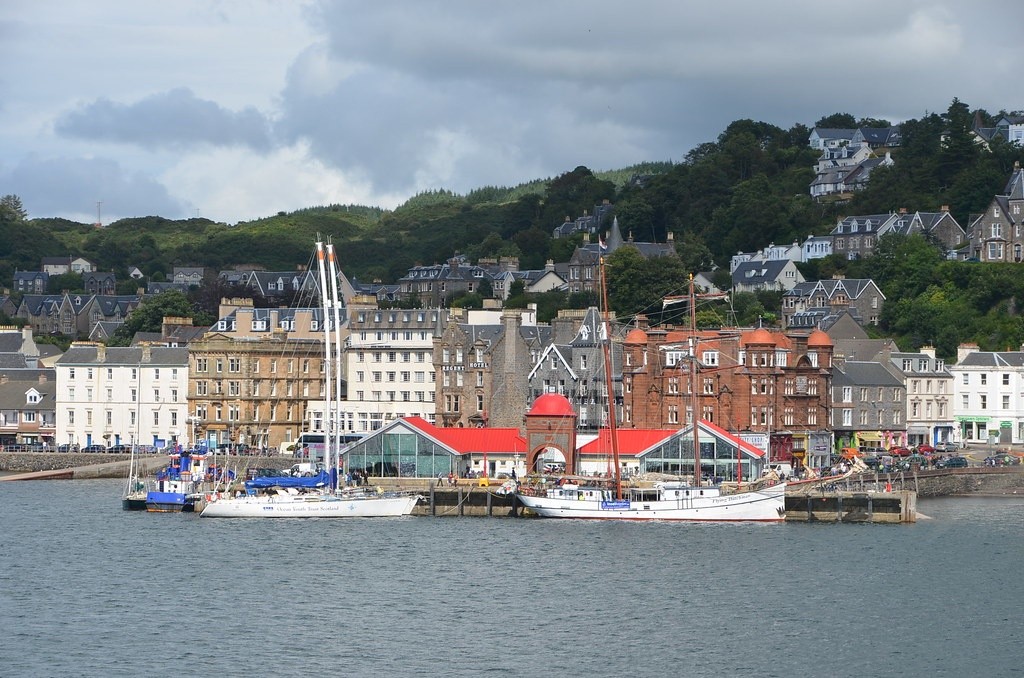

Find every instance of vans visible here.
[867,446,891,461]
[290,462,319,477]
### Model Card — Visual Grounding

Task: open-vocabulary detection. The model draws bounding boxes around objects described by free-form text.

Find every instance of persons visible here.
[77,443,80,453]
[437,471,443,487]
[43,440,50,452]
[879,462,889,473]
[447,472,458,487]
[466,464,470,473]
[292,447,308,458]
[905,462,910,471]
[512,467,515,477]
[831,458,855,475]
[780,472,785,483]
[984,457,996,467]
[347,470,369,486]
[1000,459,1005,467]
[1005,455,1010,466]
[549,465,561,474]
[931,453,958,466]
[291,468,294,476]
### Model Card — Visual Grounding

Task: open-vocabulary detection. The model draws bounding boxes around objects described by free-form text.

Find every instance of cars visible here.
[888,441,968,468]
[842,447,863,459]
[985,453,1021,466]
[4,441,129,453]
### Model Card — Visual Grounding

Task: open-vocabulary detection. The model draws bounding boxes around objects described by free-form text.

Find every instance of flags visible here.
[599,239,607,253]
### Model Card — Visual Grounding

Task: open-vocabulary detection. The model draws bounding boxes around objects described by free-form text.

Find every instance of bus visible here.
[293,432,369,459]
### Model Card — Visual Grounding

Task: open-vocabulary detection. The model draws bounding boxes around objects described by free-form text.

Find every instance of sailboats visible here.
[122,231,424,519]
[515,233,786,522]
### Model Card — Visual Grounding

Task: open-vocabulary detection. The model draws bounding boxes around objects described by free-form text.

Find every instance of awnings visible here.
[861,434,883,441]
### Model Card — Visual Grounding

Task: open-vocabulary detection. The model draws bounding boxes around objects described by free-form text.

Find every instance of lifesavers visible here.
[701,491,703,495]
[559,491,563,495]
[573,480,578,485]
[686,491,689,495]
[207,495,211,501]
[886,484,891,492]
[675,491,679,496]
